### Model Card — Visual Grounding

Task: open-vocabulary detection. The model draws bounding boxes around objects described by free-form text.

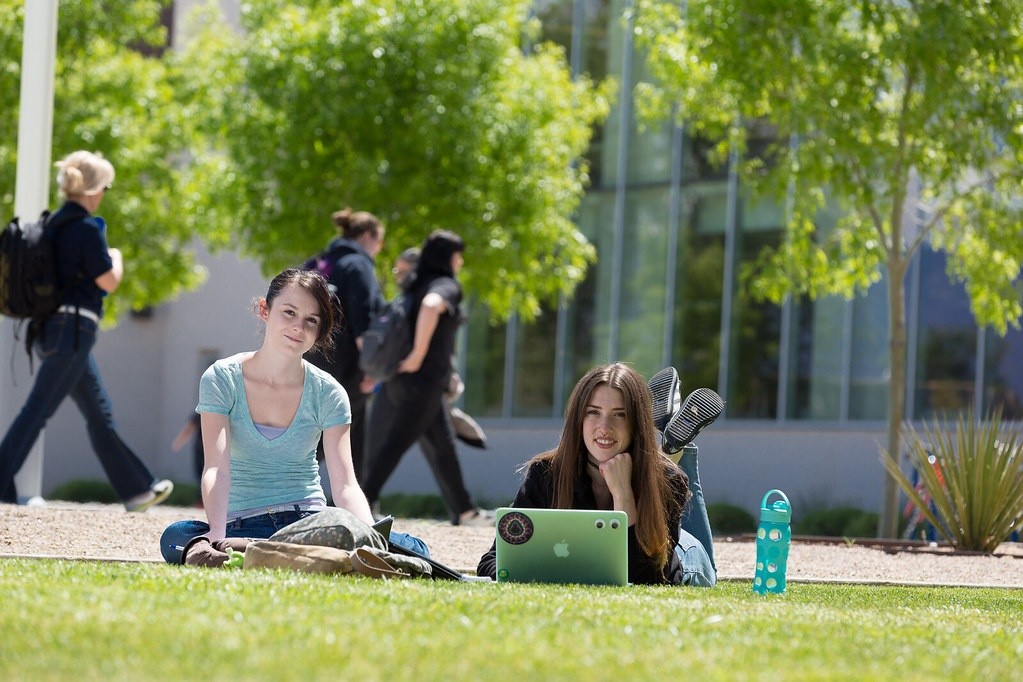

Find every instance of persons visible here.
[161,270,432,566]
[478,363,726,586]
[307,209,503,526]
[0,151,175,512]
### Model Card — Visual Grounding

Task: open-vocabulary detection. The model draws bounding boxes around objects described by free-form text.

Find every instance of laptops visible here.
[495,507,634,587]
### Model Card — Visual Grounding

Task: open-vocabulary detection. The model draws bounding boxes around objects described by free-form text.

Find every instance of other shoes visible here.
[123,479,174,512]
[661,388,724,455]
[460,507,497,527]
[648,366,681,432]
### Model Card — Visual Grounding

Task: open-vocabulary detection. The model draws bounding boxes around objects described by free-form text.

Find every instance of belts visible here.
[58,305,100,326]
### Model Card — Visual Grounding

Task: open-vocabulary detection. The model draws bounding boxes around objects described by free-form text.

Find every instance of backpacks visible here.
[301,251,357,280]
[0,209,88,319]
[357,289,411,373]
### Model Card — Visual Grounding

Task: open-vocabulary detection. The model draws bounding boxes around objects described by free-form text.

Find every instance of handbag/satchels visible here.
[242,507,432,578]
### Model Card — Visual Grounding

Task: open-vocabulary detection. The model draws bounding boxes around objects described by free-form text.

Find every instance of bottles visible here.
[753,489,793,596]
